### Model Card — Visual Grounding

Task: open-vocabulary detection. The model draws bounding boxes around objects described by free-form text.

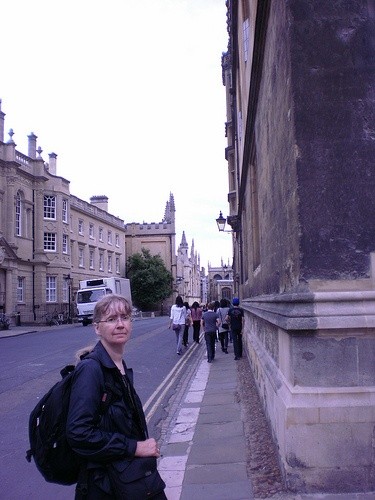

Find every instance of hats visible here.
[233,298,239,303]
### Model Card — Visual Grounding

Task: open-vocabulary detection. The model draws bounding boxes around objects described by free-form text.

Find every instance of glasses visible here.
[96,315,131,323]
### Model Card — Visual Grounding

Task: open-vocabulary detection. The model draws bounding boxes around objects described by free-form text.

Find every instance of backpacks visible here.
[29,352,114,486]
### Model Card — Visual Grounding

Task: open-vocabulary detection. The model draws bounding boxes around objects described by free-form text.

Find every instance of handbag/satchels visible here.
[172,324,179,330]
[115,456,168,500]
[222,324,229,330]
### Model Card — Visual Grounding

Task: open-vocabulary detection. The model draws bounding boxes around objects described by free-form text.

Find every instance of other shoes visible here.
[234,356,240,360]
[225,351,228,354]
[177,351,183,355]
[208,360,211,363]
[184,344,187,348]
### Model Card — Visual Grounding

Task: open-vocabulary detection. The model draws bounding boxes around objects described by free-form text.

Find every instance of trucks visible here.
[75,277,132,327]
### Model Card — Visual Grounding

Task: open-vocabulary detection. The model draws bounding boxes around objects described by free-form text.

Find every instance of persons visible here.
[63,294,169,500]
[190,302,202,344]
[216,299,230,354]
[183,302,193,347]
[201,302,221,363]
[225,297,244,360]
[214,301,220,312]
[168,296,187,355]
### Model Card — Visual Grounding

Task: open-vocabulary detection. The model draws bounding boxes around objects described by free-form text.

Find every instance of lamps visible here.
[216,211,238,233]
